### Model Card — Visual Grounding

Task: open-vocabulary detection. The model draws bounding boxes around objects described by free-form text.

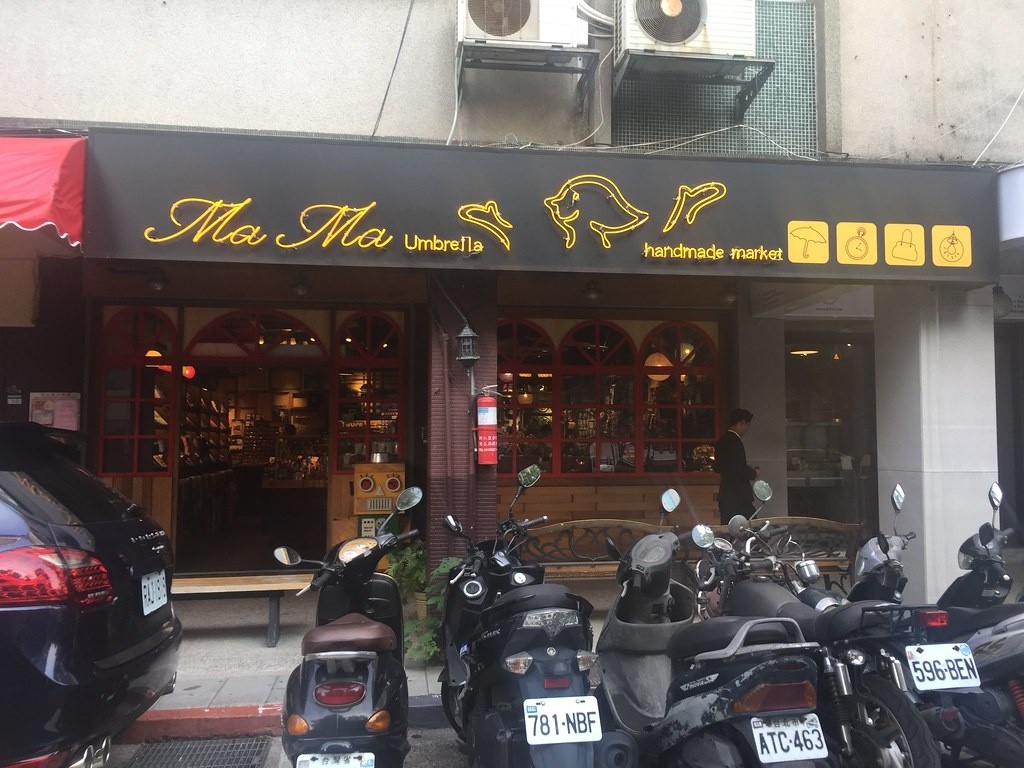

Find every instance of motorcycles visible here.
[687,479,941,768]
[437,465,604,768]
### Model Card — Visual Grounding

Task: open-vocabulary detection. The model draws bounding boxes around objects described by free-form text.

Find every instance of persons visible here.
[532,424,573,472]
[714,409,762,524]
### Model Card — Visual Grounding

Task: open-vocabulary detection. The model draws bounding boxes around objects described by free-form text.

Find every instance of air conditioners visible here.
[454,0,578,63]
[613,0,757,76]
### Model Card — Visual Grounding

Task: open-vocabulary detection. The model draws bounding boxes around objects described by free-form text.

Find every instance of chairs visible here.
[590,441,620,472]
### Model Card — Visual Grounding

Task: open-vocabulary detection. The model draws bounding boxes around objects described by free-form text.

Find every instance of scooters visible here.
[842,480,1024,767]
[273,485,423,768]
[592,488,832,768]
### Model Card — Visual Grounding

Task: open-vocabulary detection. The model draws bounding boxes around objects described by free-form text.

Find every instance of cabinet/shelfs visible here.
[146,371,231,548]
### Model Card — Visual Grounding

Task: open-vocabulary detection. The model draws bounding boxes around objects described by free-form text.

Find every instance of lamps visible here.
[235,367,328,459]
[456,321,482,377]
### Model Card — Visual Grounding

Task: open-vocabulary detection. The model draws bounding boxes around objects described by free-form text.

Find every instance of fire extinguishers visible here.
[468,385,503,464]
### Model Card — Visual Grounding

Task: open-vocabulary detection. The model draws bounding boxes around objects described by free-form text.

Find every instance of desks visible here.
[650,449,678,472]
[787,468,848,522]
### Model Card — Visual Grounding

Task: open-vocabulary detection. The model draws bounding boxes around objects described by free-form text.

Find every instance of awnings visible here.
[0,136,88,250]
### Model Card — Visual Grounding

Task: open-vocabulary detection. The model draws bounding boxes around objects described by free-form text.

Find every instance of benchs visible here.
[505,511,864,595]
[172,572,315,647]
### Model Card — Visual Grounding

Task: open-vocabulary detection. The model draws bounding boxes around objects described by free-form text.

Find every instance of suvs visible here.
[1,417,182,768]
